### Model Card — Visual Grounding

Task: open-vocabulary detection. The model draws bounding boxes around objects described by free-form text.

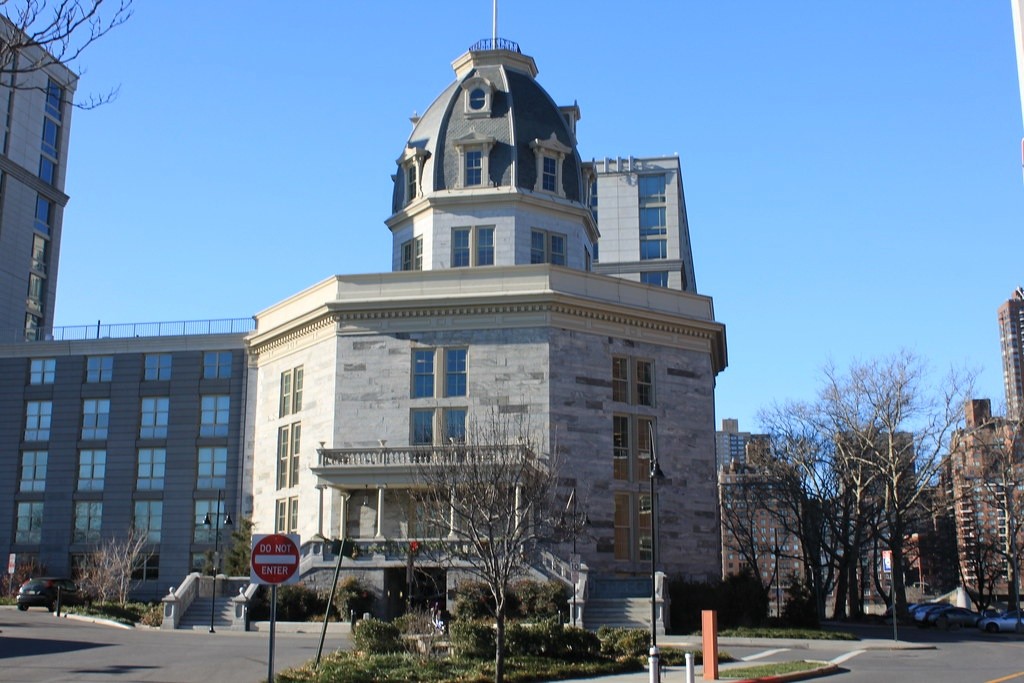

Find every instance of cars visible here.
[907,602,984,631]
[977,609,1024,634]
[16,577,92,614]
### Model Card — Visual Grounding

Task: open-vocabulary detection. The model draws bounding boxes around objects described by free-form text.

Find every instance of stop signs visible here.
[251,534,300,583]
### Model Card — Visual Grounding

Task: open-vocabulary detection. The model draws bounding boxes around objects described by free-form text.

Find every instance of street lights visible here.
[201,487,234,633]
[648,421,666,683]
[556,487,593,628]
